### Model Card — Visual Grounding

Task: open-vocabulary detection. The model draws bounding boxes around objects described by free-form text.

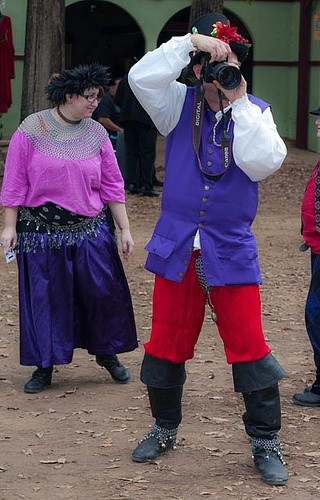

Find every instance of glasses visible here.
[79,94,101,103]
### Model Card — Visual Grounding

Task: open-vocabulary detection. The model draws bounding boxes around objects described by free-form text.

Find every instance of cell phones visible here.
[6,251,16,264]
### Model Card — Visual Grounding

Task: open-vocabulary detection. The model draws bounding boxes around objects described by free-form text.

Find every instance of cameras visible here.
[203,53,242,90]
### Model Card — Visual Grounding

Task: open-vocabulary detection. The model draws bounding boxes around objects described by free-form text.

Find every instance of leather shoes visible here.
[24,367,52,393]
[96,354,130,383]
[292,387,320,407]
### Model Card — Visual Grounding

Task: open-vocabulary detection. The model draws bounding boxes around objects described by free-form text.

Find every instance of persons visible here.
[91,55,165,197]
[128,13,289,485]
[293,105,320,406]
[0,63,138,394]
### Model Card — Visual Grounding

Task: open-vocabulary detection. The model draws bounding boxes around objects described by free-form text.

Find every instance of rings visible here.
[0,243,2,246]
[13,242,16,244]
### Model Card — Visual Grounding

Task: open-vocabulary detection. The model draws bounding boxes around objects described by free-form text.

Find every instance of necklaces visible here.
[56,106,81,125]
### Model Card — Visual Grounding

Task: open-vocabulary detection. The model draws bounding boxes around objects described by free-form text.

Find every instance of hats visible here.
[187,12,248,76]
[308,107,320,116]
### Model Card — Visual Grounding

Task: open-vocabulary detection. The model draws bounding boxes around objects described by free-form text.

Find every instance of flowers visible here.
[210,20,249,43]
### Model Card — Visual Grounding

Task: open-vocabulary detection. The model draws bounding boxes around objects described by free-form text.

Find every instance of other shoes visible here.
[127,184,138,194]
[153,181,164,187]
[132,424,178,461]
[142,186,159,197]
[250,437,289,486]
